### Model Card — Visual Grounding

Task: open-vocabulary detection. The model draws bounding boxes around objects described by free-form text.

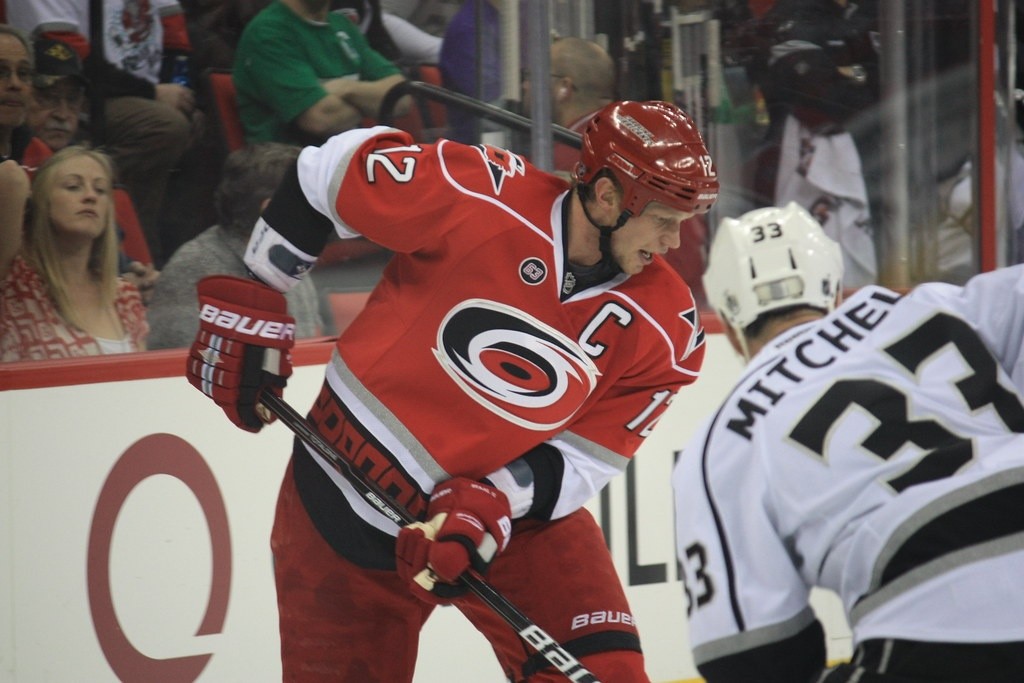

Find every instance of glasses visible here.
[520,66,578,92]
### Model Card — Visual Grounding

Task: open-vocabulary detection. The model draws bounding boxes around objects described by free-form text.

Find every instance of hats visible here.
[36,38,93,89]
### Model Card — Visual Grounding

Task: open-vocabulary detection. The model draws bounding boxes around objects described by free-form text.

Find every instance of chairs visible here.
[113,184,153,265]
[201,65,247,151]
[321,286,377,335]
[361,59,447,144]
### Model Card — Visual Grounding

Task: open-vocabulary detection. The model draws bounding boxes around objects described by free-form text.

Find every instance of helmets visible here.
[700,200,845,328]
[575,99,720,221]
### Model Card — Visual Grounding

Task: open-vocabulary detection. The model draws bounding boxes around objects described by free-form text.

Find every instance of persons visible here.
[146,142,325,349]
[0,0,215,303]
[0,146,149,363]
[184,100,720,683]
[670,200,1024,683]
[230,0,711,312]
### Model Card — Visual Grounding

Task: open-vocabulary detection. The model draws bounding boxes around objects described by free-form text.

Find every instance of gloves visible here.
[396,477,513,608]
[184,276,296,433]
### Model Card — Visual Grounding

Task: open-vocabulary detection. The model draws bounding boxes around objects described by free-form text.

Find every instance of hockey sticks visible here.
[257,387,604,683]
